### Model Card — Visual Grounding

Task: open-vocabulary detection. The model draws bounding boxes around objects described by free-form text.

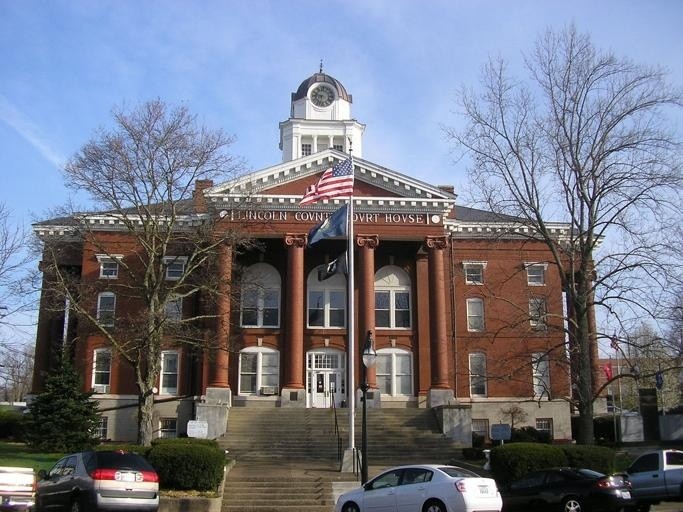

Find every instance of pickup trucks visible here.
[626,449,683,511]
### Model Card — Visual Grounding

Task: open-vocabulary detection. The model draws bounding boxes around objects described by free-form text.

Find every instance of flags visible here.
[610,334,618,351]
[318,252,349,281]
[299,156,353,207]
[602,363,612,381]
[656,373,663,391]
[308,204,348,245]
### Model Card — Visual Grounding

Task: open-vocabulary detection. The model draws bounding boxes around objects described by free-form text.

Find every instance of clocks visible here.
[307,82,338,109]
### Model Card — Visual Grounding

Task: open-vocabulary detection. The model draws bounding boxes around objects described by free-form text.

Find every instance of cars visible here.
[0,466,34,512]
[336,464,503,512]
[501,467,637,512]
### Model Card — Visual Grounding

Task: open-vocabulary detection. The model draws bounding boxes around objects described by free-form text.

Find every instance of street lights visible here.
[361,331,377,485]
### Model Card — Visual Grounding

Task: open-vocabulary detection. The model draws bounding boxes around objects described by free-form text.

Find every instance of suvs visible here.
[34,450,159,512]
